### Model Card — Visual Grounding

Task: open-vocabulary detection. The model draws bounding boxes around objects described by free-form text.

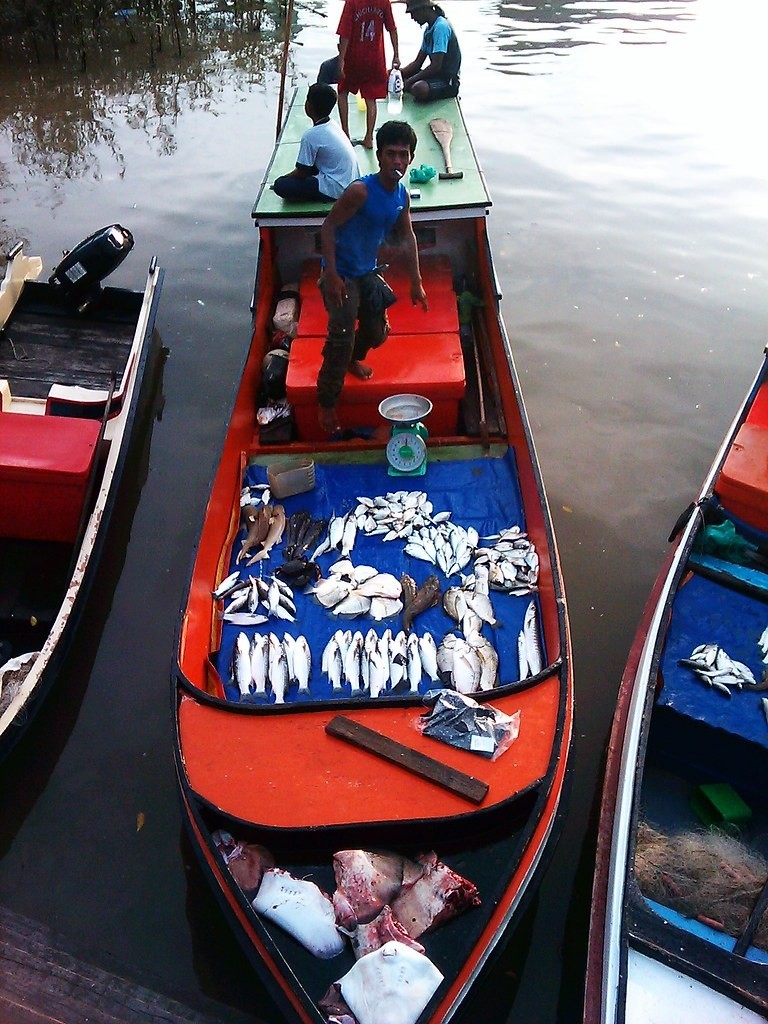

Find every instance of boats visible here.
[582,341,767,1023]
[170,80,574,1023]
[0,222,164,781]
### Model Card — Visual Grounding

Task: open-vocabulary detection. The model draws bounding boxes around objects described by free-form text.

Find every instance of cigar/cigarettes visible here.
[396,168,402,177]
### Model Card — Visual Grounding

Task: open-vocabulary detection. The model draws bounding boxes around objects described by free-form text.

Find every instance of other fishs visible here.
[210,485,542,1024]
[677,625,768,726]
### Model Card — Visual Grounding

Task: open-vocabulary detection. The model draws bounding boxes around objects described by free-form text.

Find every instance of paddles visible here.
[428,117,464,180]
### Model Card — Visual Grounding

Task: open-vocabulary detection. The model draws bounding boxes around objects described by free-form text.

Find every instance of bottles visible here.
[387,64,404,114]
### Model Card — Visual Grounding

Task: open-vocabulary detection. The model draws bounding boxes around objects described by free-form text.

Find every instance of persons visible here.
[317,120,428,432]
[389,0,461,100]
[274,82,361,200]
[337,0,401,148]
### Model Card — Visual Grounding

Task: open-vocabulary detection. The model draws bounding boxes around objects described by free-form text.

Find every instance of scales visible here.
[379,394,434,477]
